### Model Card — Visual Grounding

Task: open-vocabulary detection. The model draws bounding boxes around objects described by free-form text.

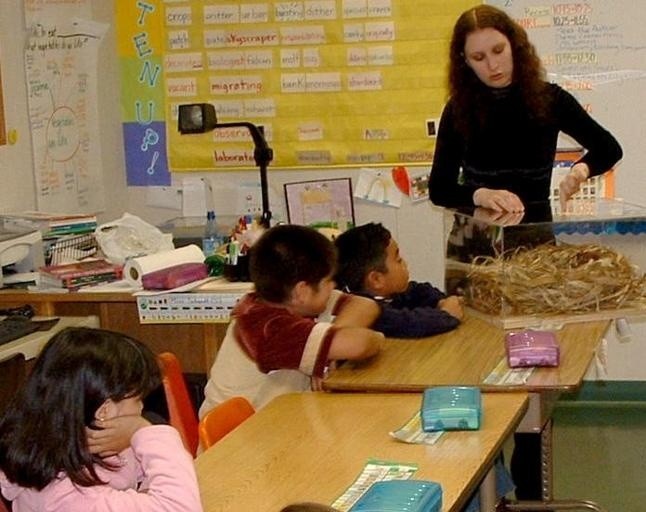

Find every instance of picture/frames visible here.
[282,177,355,242]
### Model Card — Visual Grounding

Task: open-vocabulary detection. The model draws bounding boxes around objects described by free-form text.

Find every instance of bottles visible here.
[202,212,222,257]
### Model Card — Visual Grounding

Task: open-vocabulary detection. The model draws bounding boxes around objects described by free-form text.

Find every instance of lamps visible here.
[176,101,274,282]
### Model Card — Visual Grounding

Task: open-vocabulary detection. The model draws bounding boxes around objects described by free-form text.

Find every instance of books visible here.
[3,212,123,289]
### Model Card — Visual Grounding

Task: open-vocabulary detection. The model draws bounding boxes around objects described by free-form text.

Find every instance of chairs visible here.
[154,352,256,449]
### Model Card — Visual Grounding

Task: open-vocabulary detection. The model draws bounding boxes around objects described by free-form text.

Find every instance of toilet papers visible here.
[121,243,206,288]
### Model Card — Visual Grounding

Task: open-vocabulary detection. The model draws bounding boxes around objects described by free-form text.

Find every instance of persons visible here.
[330,223,465,338]
[429,5,623,501]
[0,327,203,512]
[198,225,386,416]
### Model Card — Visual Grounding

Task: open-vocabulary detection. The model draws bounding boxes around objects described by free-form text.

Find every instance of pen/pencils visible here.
[235,215,253,235]
[226,239,239,265]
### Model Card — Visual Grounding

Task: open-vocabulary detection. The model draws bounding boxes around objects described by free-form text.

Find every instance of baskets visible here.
[44,230,99,266]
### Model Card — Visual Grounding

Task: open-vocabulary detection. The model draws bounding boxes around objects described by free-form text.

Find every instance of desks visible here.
[1,291,229,381]
[322,311,613,510]
[194,393,531,511]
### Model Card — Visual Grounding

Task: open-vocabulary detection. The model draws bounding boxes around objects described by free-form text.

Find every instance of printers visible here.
[0,222,45,289]
